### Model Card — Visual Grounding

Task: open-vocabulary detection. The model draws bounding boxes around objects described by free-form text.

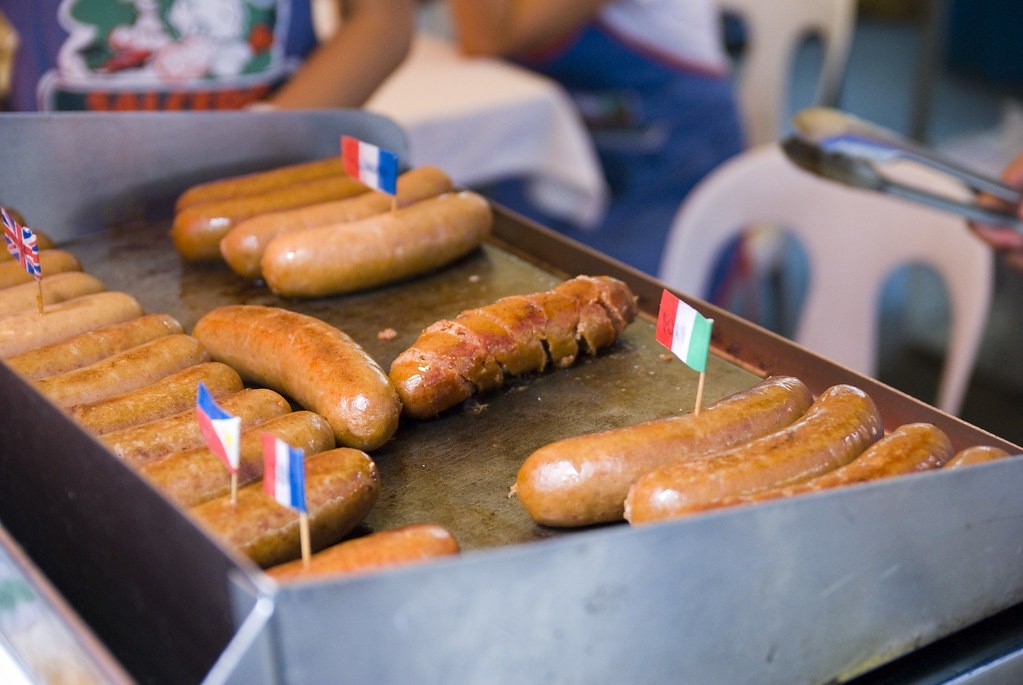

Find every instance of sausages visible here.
[0,203,460,582]
[389,276,638,419]
[168,153,494,296]
[506,372,1012,528]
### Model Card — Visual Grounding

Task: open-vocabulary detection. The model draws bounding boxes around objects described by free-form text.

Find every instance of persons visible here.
[1,0,414,112]
[451,0,739,277]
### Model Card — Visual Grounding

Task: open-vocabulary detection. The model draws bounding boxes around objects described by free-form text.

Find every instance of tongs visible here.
[818,134,1023,235]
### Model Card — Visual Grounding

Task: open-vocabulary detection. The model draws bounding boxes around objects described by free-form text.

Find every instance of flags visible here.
[197,384,242,472]
[0,206,42,280]
[657,288,713,372]
[341,136,397,195]
[261,435,307,512]
